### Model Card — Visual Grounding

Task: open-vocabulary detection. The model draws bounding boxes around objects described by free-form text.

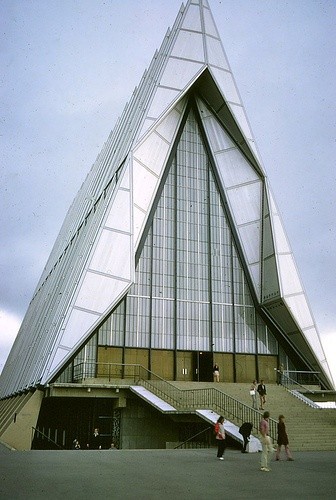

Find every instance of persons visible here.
[257,380,267,410]
[108,443,117,450]
[215,416,226,461]
[259,410,274,472]
[275,415,296,461]
[250,380,261,410]
[87,428,102,450]
[73,439,81,450]
[213,363,220,382]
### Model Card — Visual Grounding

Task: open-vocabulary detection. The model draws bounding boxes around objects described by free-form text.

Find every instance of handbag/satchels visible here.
[212,424,219,436]
[249,390,256,396]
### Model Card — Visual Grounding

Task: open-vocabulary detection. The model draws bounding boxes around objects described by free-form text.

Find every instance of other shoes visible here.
[286,457,295,461]
[240,450,249,454]
[275,457,281,460]
[260,466,270,472]
[217,457,225,460]
